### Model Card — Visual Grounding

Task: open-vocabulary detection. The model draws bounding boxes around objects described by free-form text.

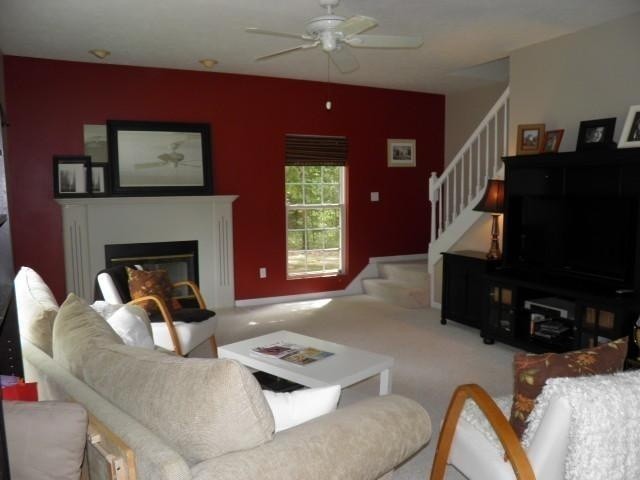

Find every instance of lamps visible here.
[316,33,341,112]
[471,178,504,261]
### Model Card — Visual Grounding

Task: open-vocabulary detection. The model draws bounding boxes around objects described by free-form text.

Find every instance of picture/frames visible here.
[386,138,417,167]
[515,103,640,155]
[52,116,214,198]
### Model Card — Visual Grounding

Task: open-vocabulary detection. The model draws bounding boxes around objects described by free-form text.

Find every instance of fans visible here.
[243,0,426,75]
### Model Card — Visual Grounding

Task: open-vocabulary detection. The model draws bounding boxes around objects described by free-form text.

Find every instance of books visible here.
[249,339,306,360]
[533,318,569,339]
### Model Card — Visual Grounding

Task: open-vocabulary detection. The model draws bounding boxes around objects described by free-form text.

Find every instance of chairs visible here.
[430,368,640,477]
[96,264,218,358]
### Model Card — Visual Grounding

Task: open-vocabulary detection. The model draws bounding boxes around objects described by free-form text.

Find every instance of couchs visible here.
[19,299,433,479]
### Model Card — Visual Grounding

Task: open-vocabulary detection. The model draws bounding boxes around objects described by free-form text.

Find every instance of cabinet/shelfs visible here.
[438,250,501,334]
[482,272,635,357]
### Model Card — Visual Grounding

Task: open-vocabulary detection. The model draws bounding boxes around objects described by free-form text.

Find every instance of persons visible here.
[583,126,605,142]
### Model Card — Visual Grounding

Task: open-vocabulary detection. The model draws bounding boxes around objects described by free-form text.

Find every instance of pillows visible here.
[503,334,630,464]
[2,400,89,478]
